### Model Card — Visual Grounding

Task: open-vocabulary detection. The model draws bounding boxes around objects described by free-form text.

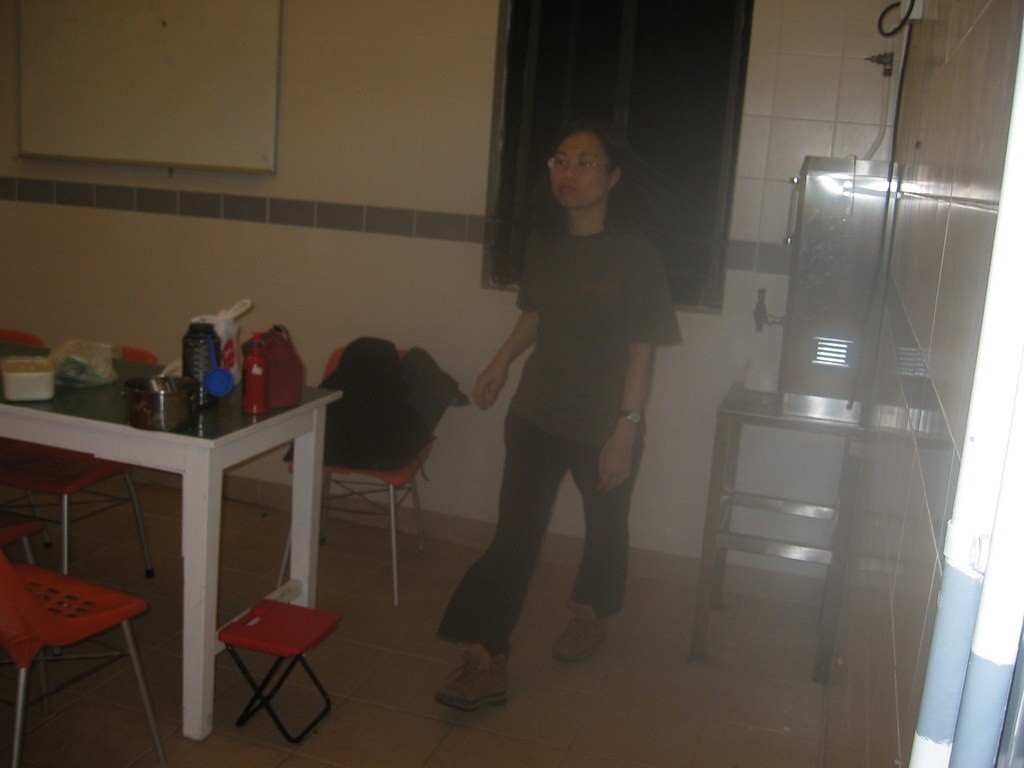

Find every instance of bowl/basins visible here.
[123,374,198,430]
[0,354,61,402]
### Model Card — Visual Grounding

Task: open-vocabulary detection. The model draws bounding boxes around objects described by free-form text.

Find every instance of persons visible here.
[435,119,682,712]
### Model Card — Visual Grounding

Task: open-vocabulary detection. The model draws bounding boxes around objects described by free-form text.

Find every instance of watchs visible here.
[620,410,642,424]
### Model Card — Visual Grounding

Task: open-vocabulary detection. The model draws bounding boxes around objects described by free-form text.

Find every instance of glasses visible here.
[548,158,612,172]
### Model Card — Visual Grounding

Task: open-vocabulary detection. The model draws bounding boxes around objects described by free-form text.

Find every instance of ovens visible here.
[780,156,896,423]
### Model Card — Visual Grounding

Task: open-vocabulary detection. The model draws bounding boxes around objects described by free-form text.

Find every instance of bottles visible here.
[241,332,269,415]
[182,321,234,409]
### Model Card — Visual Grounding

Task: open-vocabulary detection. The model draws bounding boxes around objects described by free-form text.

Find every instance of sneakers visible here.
[552,601,609,661]
[434,654,508,711]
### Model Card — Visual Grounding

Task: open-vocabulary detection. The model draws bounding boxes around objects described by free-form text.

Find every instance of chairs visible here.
[0,349,432,768]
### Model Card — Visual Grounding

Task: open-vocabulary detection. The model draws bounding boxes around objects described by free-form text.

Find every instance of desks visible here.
[1,338,341,743]
[689,381,874,682]
